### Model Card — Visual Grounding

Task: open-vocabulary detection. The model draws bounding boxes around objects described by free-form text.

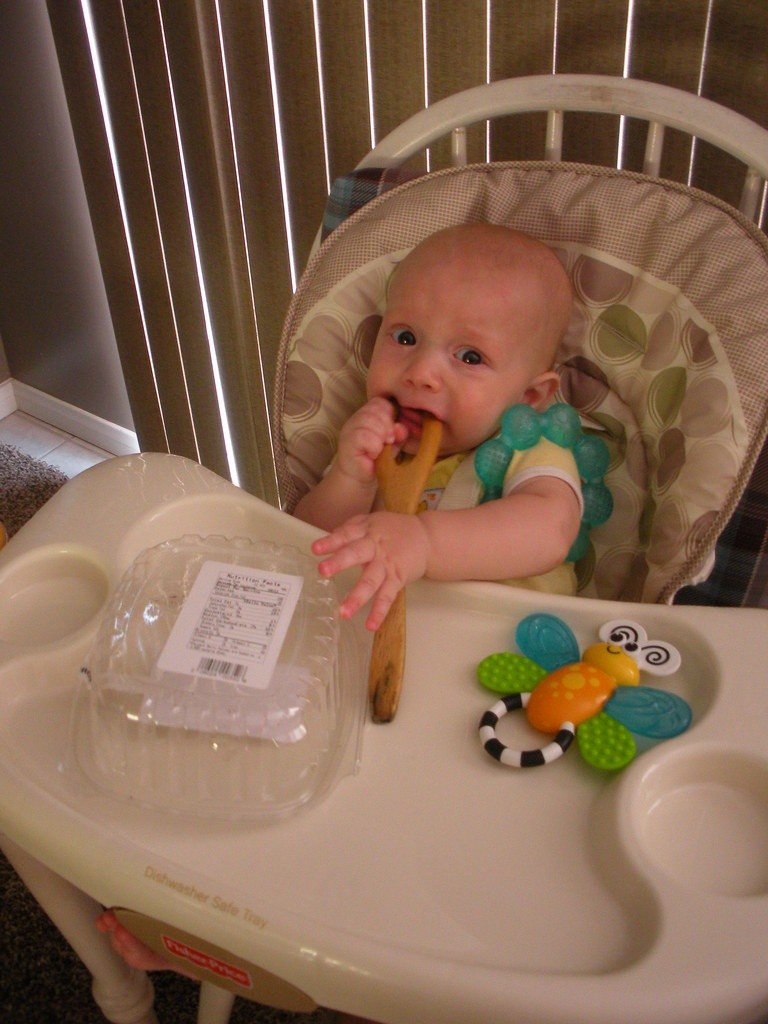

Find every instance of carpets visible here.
[1,440,380,1023]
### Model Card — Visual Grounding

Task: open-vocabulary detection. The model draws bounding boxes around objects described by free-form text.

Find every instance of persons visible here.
[291,223,584,633]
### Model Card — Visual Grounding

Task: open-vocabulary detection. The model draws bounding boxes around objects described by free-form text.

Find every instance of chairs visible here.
[0,74,768,1023]
